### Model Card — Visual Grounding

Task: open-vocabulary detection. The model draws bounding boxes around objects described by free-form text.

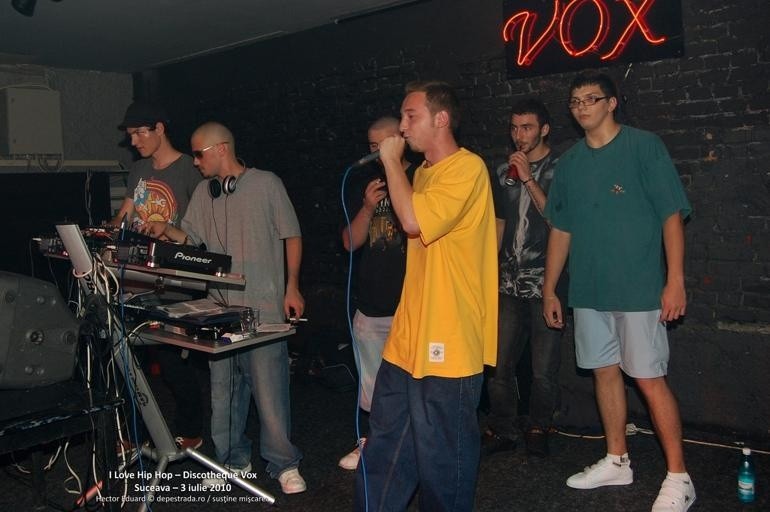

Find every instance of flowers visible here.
[355,140,407,167]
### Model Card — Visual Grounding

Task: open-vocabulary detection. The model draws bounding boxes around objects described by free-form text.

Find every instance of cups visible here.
[567,96,609,109]
[193,142,229,160]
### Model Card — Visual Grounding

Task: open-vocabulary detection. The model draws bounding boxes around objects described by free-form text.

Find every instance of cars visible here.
[239,308,260,334]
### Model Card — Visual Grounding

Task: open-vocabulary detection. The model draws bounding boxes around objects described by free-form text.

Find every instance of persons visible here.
[354,70,500,511]
[479,99,567,458]
[133,121,306,495]
[543,71,698,511]
[339,115,420,470]
[113,101,204,457]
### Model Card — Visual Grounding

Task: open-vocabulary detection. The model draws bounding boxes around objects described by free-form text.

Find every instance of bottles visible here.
[117,102,171,131]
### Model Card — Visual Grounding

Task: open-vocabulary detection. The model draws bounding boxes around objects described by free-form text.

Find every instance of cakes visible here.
[505,163,518,186]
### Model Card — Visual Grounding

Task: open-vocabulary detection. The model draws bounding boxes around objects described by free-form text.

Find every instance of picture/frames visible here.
[0,383,125,512]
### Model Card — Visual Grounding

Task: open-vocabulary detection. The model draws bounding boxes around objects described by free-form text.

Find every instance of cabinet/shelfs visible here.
[505,153,519,188]
[735,446,758,504]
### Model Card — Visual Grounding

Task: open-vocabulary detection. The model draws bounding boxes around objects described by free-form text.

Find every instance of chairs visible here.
[480,427,517,454]
[566,456,634,489]
[338,436,367,470]
[524,423,551,459]
[278,466,307,495]
[201,460,253,488]
[116,438,150,457]
[651,475,698,511]
[175,436,203,449]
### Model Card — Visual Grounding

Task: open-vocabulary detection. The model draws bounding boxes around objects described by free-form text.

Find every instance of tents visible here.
[207,155,246,199]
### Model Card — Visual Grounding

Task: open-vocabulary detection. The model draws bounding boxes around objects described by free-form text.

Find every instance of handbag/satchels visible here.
[522,177,535,185]
